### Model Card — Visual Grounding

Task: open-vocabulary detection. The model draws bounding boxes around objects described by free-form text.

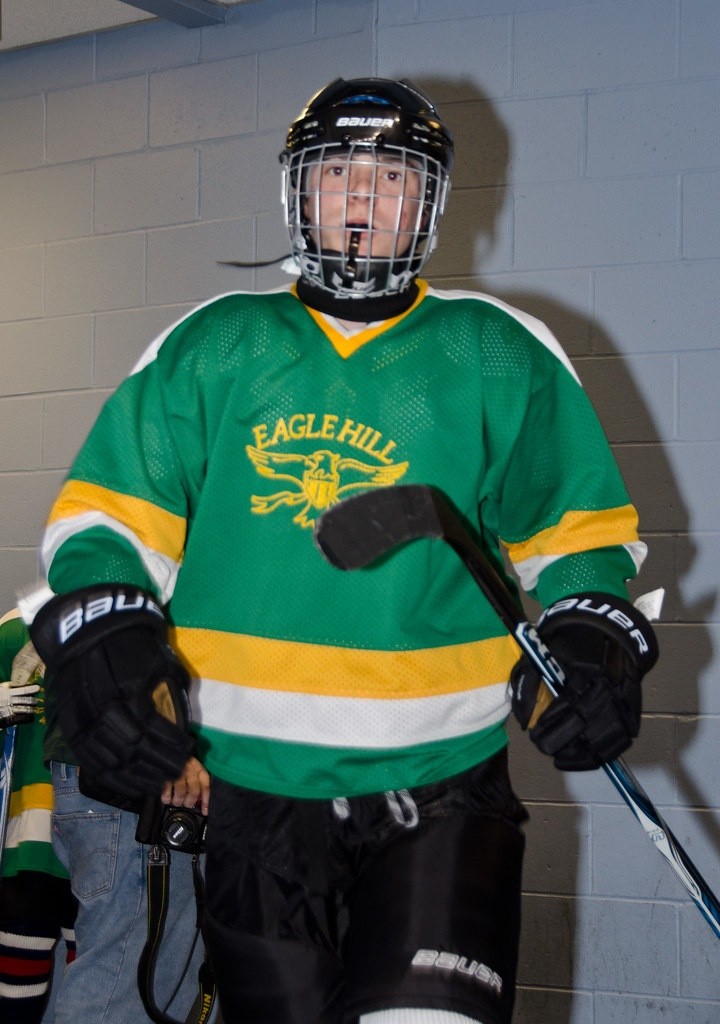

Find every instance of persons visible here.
[20,74,660,1024]
[0,582,206,1024]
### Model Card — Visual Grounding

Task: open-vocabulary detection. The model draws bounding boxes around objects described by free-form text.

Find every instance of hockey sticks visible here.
[314,482,720,941]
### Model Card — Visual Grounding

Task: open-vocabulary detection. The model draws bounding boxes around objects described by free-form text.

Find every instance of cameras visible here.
[134,800,210,854]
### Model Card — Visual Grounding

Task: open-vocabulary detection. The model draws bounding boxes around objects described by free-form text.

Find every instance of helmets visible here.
[278,77,454,320]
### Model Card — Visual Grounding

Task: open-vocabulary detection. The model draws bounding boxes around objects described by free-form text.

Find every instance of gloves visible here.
[506,592,660,772]
[0,682,40,721]
